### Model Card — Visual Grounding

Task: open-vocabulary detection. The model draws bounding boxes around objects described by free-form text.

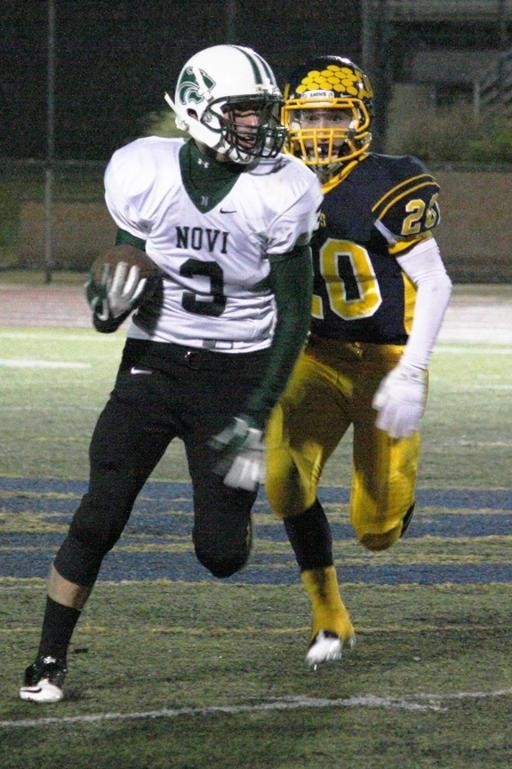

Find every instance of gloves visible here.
[85,261,148,333]
[372,364,427,438]
[211,412,268,490]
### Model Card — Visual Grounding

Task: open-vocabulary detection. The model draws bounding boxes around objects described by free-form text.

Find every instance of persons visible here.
[19,43,324,705]
[264,54,453,664]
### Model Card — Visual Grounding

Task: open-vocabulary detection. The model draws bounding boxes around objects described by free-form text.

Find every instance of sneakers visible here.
[305,629,356,666]
[20,654,68,702]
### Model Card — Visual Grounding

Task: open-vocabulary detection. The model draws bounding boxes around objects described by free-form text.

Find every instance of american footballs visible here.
[92,246,161,299]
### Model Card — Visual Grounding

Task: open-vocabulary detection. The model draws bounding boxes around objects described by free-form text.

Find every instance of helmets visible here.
[175,44,288,165]
[280,55,374,166]
